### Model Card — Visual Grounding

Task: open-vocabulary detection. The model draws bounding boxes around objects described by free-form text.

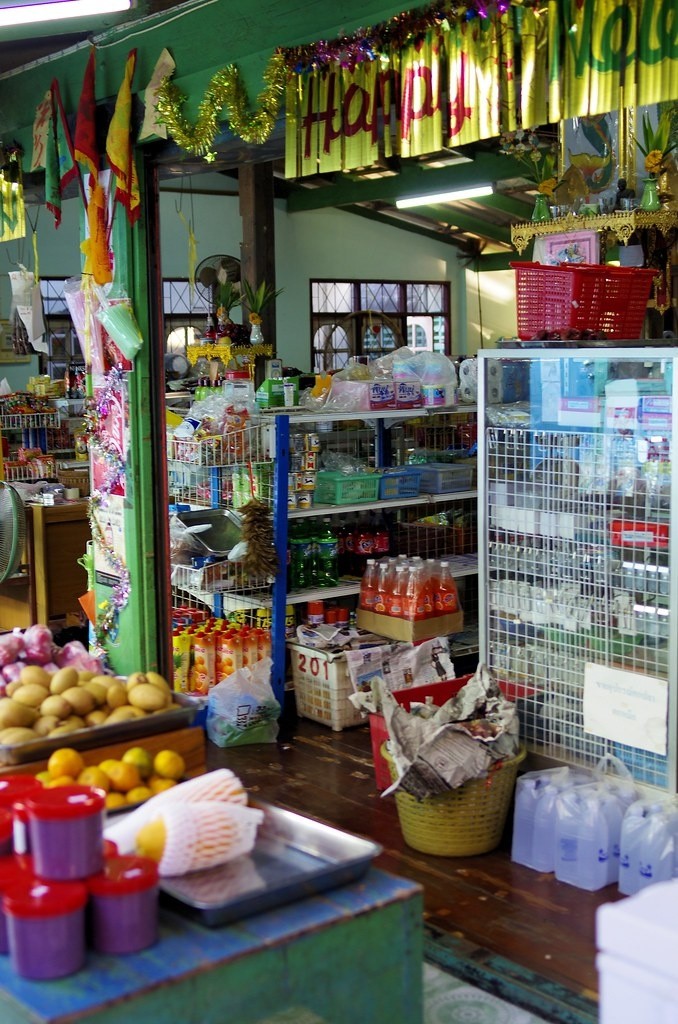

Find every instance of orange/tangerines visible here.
[193,653,262,695]
[35,746,183,807]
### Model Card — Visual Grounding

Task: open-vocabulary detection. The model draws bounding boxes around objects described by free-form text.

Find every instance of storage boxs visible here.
[364,672,536,790]
[353,605,462,643]
[289,635,448,730]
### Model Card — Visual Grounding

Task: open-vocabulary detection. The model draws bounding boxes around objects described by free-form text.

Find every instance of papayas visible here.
[136,774,250,875]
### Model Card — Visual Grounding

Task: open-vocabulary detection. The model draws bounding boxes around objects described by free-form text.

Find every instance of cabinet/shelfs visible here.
[40,313,84,398]
[161,401,479,730]
[26,495,88,632]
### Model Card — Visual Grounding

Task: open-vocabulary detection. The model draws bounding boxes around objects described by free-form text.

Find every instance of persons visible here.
[613,179,635,211]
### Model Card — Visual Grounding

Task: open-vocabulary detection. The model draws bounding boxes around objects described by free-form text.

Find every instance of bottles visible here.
[509,769,678,895]
[0,774,159,978]
[195,377,225,401]
[286,509,457,641]
[283,383,293,407]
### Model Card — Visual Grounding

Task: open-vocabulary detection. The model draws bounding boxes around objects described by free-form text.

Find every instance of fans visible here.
[0,478,25,584]
[194,253,244,324]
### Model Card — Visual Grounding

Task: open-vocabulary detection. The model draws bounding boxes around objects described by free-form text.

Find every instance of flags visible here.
[74,44,100,191]
[29,75,80,229]
[106,48,141,228]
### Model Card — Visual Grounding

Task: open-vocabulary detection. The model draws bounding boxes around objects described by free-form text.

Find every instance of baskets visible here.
[379,740,529,857]
[509,260,659,341]
[57,469,90,497]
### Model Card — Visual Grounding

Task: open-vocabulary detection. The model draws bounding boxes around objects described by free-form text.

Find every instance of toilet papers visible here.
[459,357,530,405]
[371,346,457,407]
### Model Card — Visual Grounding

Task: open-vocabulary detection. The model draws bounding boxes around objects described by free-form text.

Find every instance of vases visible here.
[249,322,265,346]
[639,177,660,211]
[525,188,550,221]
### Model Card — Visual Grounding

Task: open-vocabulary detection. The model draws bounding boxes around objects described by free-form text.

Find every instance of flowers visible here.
[234,276,286,323]
[498,122,566,184]
[632,109,677,179]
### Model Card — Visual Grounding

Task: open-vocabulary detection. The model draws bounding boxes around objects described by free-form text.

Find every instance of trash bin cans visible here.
[380,736,527,856]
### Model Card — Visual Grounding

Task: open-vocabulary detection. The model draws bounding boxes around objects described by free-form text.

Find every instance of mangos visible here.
[0,666,183,749]
